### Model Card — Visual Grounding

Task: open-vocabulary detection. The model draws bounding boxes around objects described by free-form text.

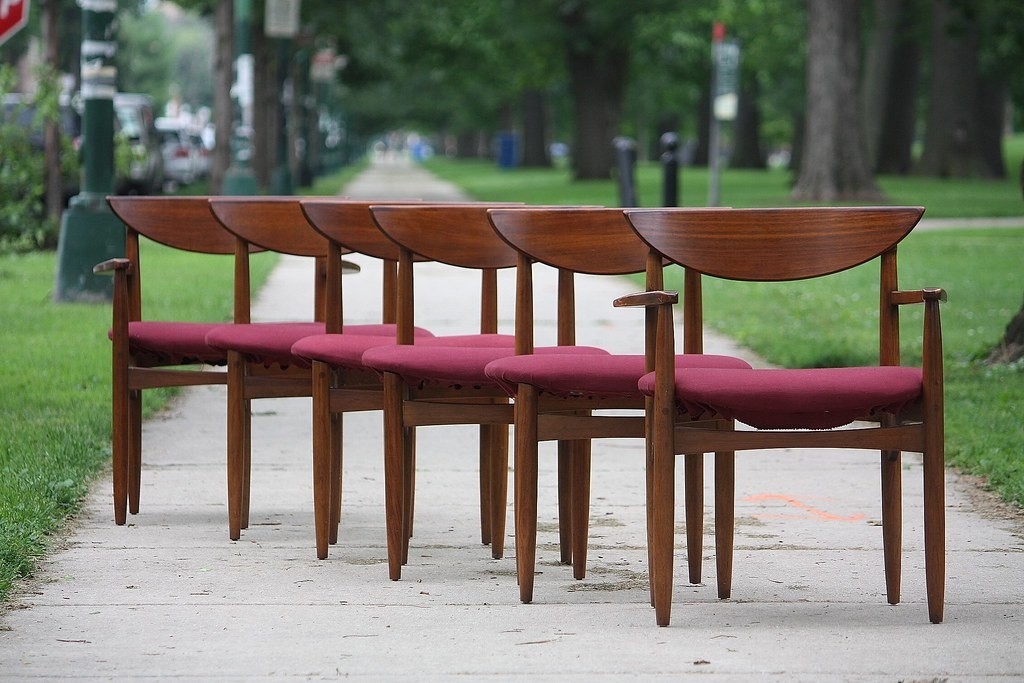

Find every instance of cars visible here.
[158,126,215,182]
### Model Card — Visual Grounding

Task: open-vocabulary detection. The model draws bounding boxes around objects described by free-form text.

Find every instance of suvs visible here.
[112,94,158,192]
[0,93,83,212]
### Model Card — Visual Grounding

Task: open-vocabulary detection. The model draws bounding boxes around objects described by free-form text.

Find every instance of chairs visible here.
[613,205,949,628]
[483,206,753,604]
[93,195,344,526]
[361,204,612,581]
[290,200,518,560]
[205,198,437,540]
